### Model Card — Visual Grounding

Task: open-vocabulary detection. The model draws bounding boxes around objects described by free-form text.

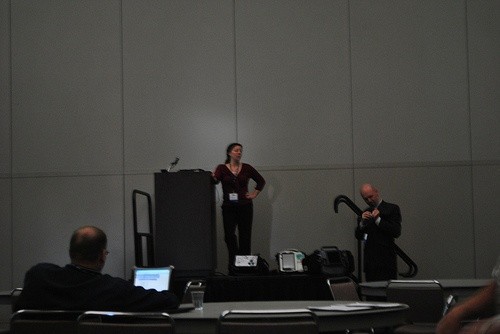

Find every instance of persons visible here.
[212,143,266,275]
[435,278,500,334]
[354,183,402,302]
[13,226,177,320]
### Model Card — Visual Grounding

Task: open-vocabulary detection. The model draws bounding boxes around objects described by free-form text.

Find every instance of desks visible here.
[167,300,416,334]
[358,278,493,323]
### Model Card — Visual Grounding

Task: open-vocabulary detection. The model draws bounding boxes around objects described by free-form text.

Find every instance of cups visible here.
[191,290,204,310]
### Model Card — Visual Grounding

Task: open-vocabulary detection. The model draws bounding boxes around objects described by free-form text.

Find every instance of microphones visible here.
[173,158,180,165]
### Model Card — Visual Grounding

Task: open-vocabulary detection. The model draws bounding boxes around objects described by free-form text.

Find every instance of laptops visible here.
[133,266,171,292]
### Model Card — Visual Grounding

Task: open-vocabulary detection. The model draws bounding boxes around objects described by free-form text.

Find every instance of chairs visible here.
[8,310,88,334]
[386,279,458,334]
[325,275,374,334]
[73,311,177,334]
